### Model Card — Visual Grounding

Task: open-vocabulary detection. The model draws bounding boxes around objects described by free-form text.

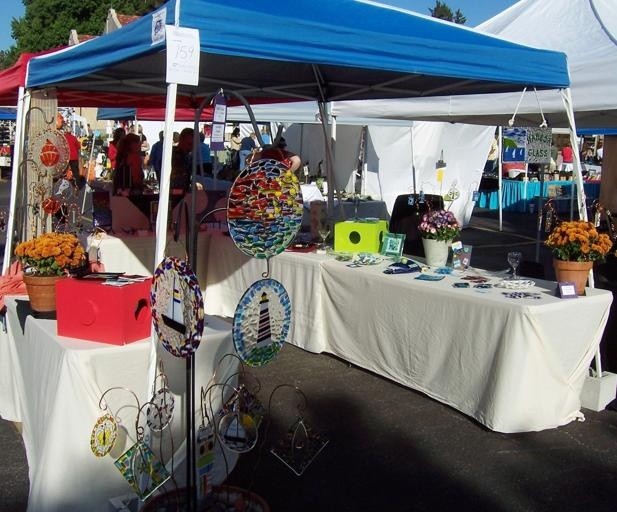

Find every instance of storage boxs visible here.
[334,220,387,254]
[56,277,153,346]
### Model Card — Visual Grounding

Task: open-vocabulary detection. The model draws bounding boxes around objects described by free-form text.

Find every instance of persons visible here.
[483,137,605,182]
[0,143,11,157]
[63,127,301,213]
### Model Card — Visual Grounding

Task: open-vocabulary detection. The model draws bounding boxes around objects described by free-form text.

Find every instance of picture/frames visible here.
[380,233,406,257]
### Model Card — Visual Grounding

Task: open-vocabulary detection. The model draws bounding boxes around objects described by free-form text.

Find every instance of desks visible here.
[302,192,391,226]
[197,226,614,433]
[477,178,600,213]
[0,273,240,512]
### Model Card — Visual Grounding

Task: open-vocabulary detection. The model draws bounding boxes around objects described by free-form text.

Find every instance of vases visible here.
[23,275,64,312]
[553,258,593,295]
[422,237,448,267]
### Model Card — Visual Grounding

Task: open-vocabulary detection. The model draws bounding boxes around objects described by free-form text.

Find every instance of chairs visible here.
[389,195,444,258]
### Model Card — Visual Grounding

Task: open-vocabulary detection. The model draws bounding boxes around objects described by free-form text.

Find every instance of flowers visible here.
[418,210,461,243]
[544,221,613,262]
[14,232,86,276]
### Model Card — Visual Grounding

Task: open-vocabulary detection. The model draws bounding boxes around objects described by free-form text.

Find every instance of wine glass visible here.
[508,252,522,280]
[317,223,330,250]
